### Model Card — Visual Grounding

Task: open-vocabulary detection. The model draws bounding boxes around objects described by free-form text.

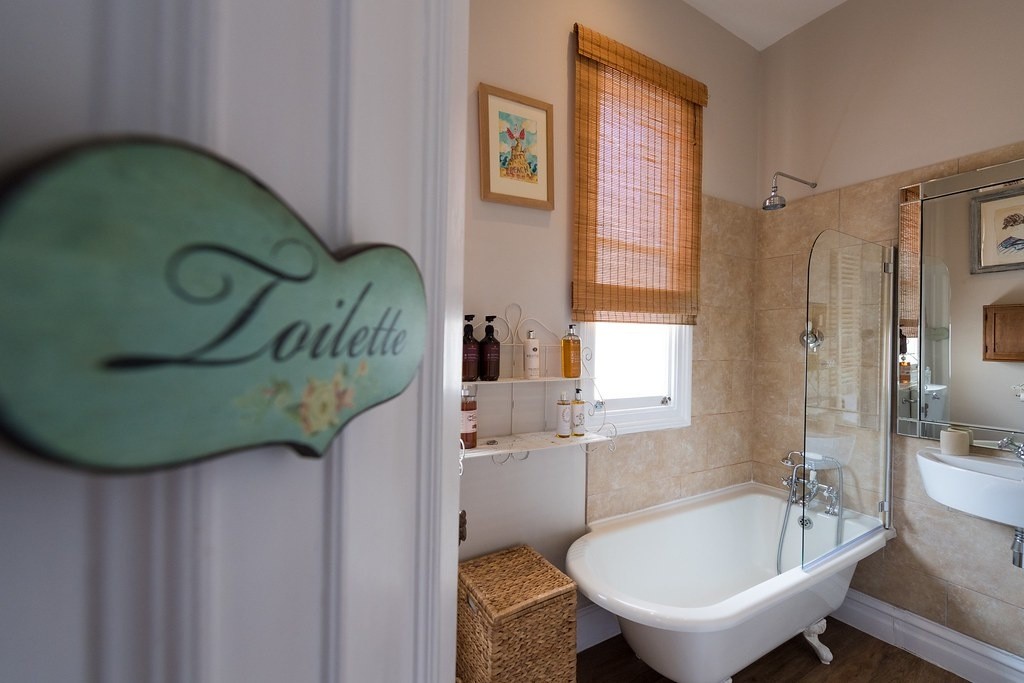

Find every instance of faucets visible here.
[798,481,820,506]
[1016,440,1024,462]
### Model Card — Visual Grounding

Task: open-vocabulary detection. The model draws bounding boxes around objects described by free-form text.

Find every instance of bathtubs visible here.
[565,480,898,683]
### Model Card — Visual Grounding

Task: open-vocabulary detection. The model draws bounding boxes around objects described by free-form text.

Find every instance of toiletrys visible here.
[461,314,480,382]
[524,330,540,379]
[555,391,572,438]
[461,385,479,450]
[571,388,586,438]
[478,315,500,381]
[899,324,932,385]
[561,323,582,378]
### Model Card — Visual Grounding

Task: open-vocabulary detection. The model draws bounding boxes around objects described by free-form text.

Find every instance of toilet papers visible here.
[940,428,970,457]
[948,426,974,445]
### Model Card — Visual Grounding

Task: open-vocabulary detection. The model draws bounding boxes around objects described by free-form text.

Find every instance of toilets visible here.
[909,384,947,423]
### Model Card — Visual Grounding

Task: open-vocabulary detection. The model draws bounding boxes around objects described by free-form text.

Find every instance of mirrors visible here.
[896,158,1024,452]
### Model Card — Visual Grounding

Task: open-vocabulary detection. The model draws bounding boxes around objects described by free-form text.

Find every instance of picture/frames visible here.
[970,183,1024,274]
[479,82,553,210]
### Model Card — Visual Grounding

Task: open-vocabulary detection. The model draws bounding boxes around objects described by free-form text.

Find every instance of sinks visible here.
[971,439,1023,451]
[916,446,1024,528]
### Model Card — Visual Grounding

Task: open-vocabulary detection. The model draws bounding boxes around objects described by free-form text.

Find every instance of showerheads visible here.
[780,450,825,468]
[762,171,818,212]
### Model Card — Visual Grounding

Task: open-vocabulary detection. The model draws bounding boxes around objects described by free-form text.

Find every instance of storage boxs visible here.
[455,543,577,683]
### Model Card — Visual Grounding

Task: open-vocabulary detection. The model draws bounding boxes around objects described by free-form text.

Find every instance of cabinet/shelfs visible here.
[459,302,617,475]
[984,304,1024,361]
